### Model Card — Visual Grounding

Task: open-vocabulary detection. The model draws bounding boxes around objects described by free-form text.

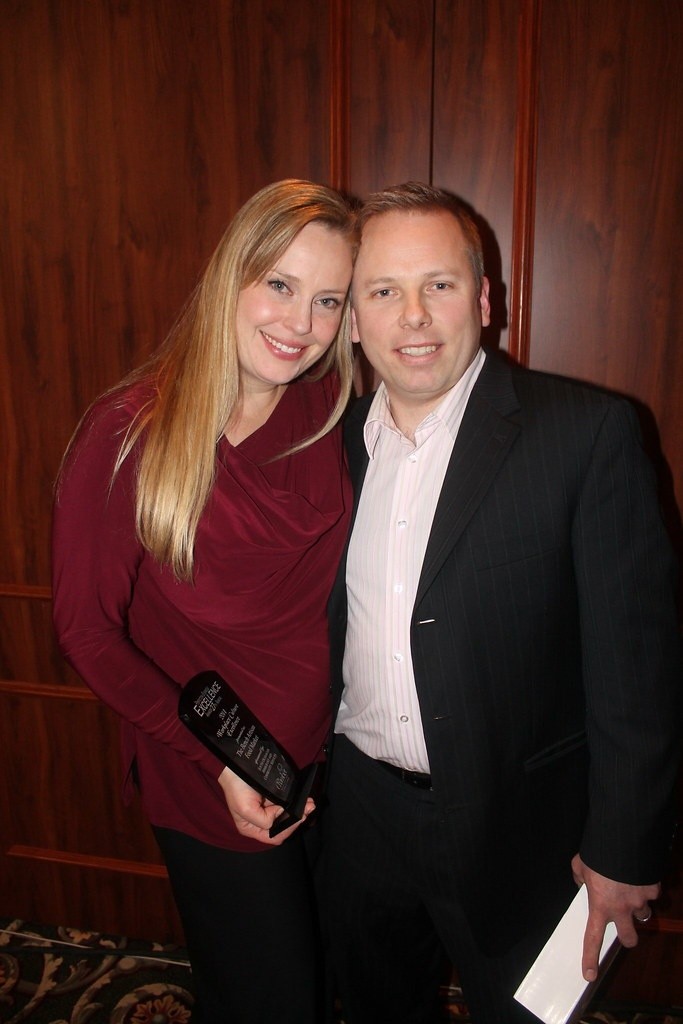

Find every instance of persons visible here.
[323,183,683,1024]
[54,179,351,1024]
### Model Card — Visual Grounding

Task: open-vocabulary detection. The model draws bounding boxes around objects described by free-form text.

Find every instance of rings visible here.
[636,908,652,922]
